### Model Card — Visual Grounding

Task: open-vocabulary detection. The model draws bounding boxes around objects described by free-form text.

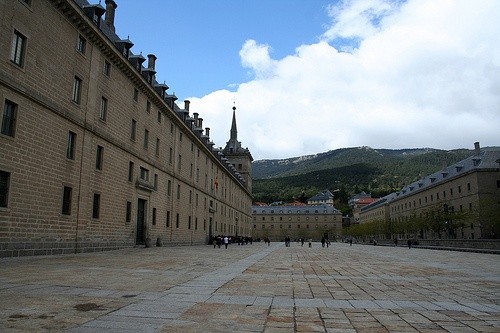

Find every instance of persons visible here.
[408,238,412,249]
[213,234,228,249]
[285,236,290,247]
[374,240,376,246]
[309,237,313,247]
[301,238,304,246]
[238,235,253,245]
[350,239,353,246]
[264,237,270,246]
[320,236,330,248]
[394,239,397,246]
